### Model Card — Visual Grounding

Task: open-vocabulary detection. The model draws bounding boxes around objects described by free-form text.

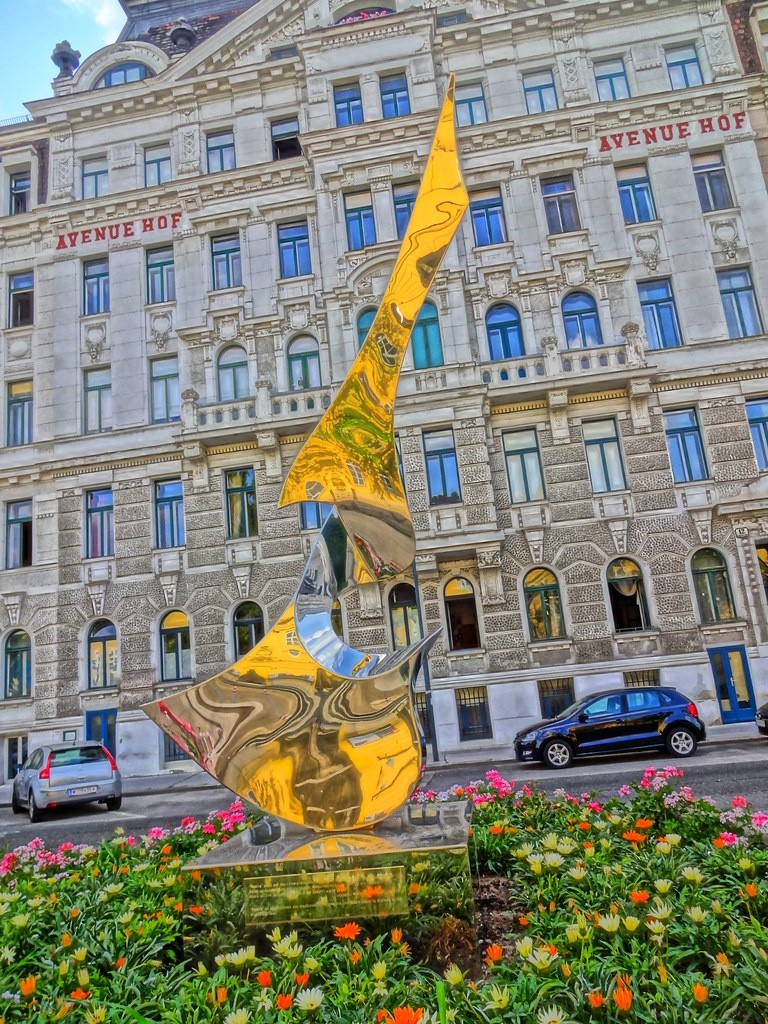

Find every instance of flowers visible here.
[339,10,386,23]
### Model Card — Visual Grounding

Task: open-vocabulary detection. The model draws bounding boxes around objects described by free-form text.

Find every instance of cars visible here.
[512,685,707,769]
[11,739,123,823]
[754,702,768,736]
